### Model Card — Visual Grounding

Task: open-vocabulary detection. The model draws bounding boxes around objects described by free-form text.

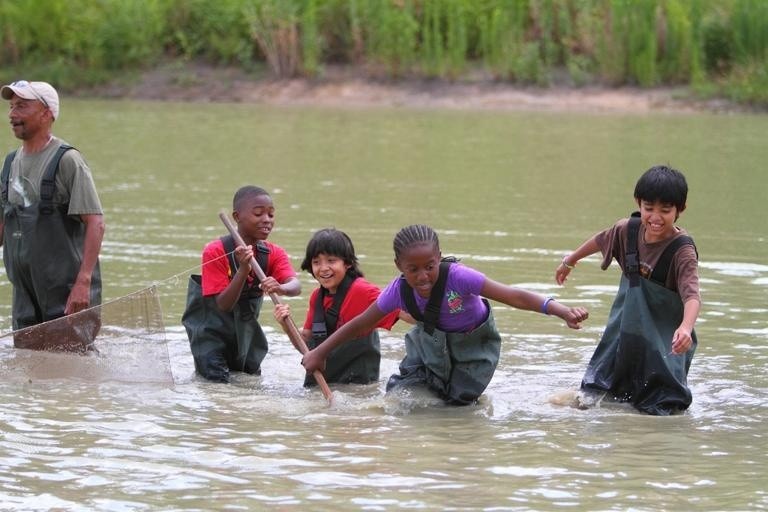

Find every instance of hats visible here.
[1,81,59,120]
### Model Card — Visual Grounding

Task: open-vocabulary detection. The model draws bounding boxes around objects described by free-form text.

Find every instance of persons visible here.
[554,164,701,418]
[300,223,590,408]
[271,226,416,390]
[0,76,108,356]
[178,185,304,387]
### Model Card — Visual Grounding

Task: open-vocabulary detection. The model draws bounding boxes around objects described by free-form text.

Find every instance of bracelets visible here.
[541,295,553,317]
[560,256,577,271]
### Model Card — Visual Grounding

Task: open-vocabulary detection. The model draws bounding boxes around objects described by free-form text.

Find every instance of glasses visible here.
[11,80,47,106]
